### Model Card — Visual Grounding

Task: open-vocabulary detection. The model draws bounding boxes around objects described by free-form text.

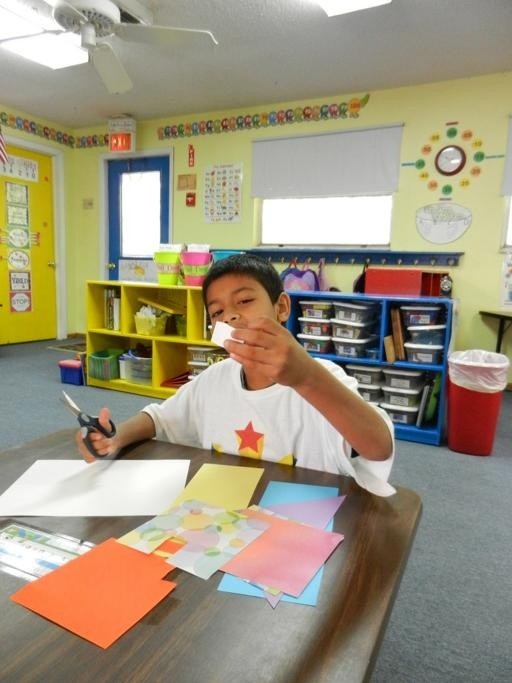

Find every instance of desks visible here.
[479,310,512,353]
[1,427,423,683]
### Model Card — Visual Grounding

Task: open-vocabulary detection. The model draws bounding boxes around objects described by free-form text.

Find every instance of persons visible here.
[75,253,398,499]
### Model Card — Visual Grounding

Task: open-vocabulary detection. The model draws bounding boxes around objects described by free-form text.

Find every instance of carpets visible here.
[44,342,86,354]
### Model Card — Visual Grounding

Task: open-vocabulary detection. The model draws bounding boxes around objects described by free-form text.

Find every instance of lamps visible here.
[80,21,96,47]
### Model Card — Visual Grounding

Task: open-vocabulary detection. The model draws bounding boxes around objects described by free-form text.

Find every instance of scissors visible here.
[59,391,116,458]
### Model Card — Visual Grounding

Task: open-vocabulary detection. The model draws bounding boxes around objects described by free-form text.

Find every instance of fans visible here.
[0,0,219,97]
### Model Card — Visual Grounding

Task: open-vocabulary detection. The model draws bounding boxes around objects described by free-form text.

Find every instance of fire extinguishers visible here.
[188,145,194,167]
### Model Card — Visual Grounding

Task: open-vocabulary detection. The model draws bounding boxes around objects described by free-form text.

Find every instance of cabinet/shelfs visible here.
[282,289,454,446]
[85,280,230,400]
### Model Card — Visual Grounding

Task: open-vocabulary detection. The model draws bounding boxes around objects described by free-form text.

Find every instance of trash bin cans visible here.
[446,349,510,456]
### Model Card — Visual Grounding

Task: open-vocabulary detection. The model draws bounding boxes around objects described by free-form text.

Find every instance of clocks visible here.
[434,145,465,176]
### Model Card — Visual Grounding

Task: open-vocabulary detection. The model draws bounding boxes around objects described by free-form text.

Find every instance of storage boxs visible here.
[180,251,212,286]
[133,313,168,337]
[211,251,245,264]
[57,359,82,385]
[296,299,379,357]
[88,346,126,381]
[119,353,152,386]
[400,304,447,364]
[345,364,426,425]
[153,250,181,285]
